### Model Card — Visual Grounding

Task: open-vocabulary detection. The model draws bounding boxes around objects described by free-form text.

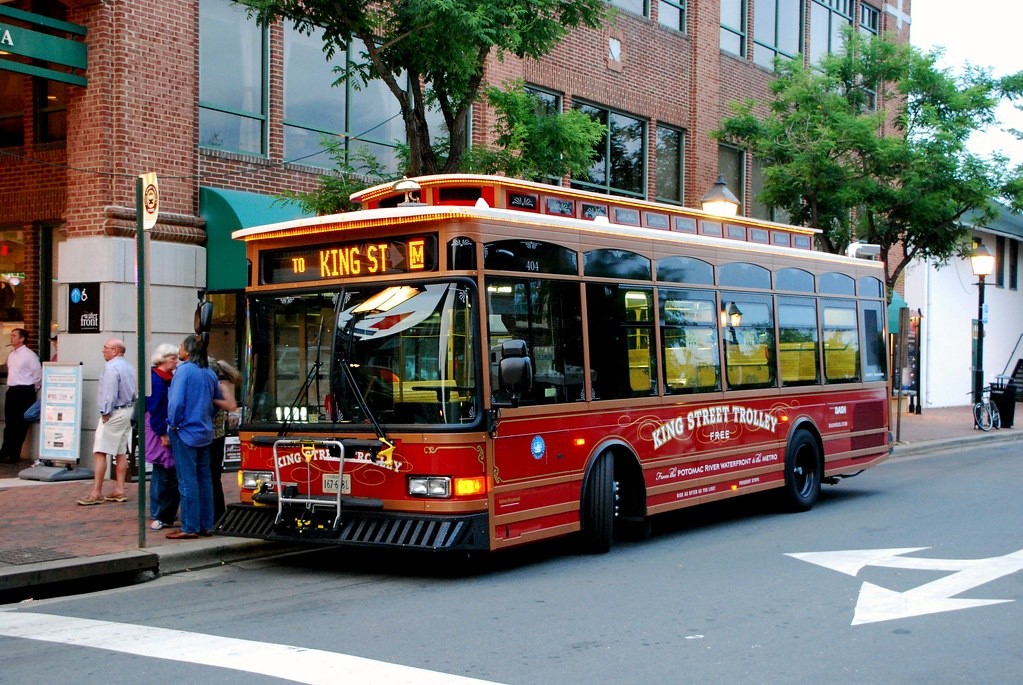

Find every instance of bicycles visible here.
[964,385,1002,432]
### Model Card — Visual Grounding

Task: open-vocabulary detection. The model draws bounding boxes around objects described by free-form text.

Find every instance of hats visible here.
[49,335,57,340]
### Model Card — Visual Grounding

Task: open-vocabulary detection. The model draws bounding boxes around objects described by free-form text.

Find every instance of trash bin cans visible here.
[988,374,1018,428]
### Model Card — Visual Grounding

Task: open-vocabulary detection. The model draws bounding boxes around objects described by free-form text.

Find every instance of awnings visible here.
[887,289,911,334]
[200,185,329,294]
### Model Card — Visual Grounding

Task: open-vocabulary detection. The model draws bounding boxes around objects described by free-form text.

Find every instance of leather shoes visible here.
[199,529,211,537]
[165,529,199,539]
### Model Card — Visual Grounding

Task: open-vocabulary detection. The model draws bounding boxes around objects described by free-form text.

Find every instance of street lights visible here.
[967,243,999,430]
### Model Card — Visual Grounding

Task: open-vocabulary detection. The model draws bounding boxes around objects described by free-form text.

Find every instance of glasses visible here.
[104,346,117,350]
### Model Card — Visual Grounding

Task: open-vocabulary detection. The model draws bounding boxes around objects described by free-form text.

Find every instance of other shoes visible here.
[151,520,162,530]
[0,456,19,463]
[31,459,44,467]
[163,521,173,526]
[77,495,104,504]
[105,492,128,501]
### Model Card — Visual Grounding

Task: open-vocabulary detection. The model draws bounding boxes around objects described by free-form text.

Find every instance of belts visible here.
[113,404,133,409]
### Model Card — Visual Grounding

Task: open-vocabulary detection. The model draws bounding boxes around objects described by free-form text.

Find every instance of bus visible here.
[195,172,897,558]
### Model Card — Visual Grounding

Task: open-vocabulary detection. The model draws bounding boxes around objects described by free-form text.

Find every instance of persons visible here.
[144,344,179,529]
[206,358,244,523]
[165,335,217,538]
[76,338,137,505]
[0,329,42,466]
